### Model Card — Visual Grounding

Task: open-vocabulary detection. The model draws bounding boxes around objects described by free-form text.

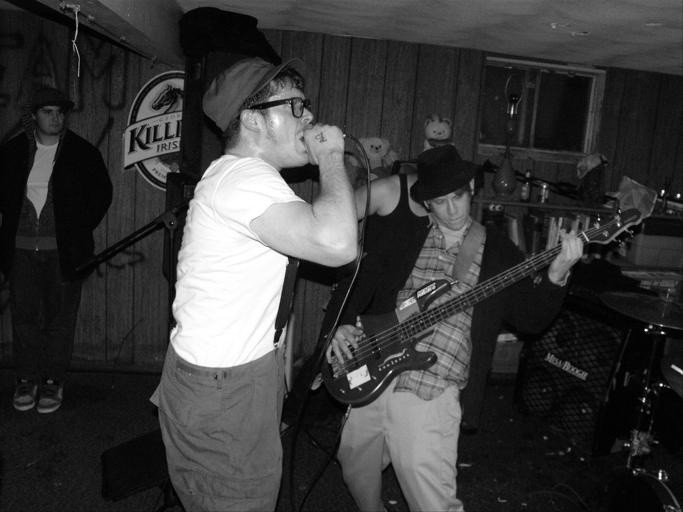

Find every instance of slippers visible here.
[236,97,312,120]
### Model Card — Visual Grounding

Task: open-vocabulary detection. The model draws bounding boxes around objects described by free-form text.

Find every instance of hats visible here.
[202,56,303,132]
[410,144,478,202]
[31,87,75,114]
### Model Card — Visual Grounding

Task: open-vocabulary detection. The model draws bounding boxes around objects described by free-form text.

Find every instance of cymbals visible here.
[661,344,682,399]
[597,290,683,331]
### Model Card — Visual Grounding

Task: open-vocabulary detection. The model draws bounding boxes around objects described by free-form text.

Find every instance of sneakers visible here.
[12,377,63,414]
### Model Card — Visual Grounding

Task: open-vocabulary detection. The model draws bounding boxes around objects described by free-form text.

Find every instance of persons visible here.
[326,145,583,512]
[149,56,357,511]
[0,87,112,415]
[282,173,433,436]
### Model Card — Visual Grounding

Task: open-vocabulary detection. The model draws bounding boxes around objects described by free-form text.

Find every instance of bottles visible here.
[521,169,532,205]
[537,182,552,204]
[493,148,517,203]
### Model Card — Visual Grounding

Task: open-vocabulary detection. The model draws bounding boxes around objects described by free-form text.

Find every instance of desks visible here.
[594,291,683,484]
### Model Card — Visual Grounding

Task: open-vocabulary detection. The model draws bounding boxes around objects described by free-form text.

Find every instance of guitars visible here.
[321,209,642,406]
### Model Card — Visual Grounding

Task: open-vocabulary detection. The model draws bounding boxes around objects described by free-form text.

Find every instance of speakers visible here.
[514,259,660,464]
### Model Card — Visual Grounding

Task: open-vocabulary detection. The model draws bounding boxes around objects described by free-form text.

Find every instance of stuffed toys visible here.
[424,117,455,153]
[350,137,391,181]
